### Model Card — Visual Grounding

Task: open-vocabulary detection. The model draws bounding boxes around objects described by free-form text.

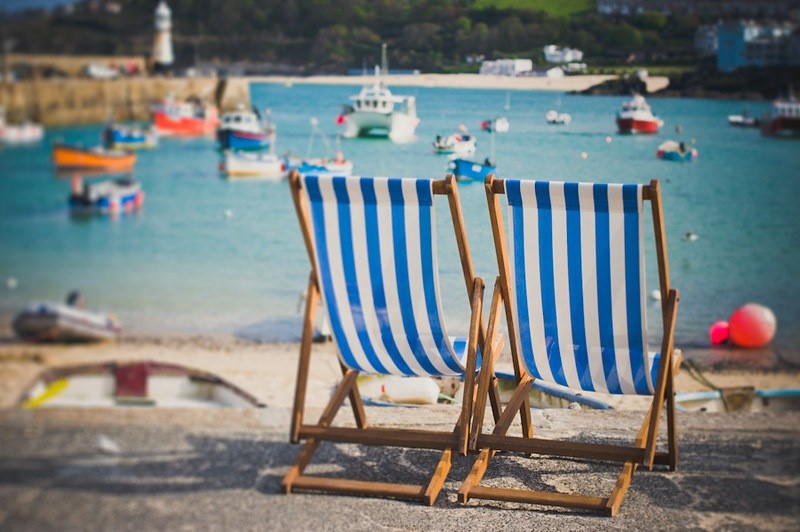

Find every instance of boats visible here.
[218,150,281,180]
[337,64,419,142]
[545,109,571,124]
[51,143,134,173]
[483,115,510,133]
[616,93,659,134]
[332,373,616,412]
[102,120,158,149]
[657,140,695,162]
[759,101,800,139]
[216,109,276,151]
[67,176,143,215]
[282,117,352,178]
[12,288,121,342]
[447,155,495,182]
[0,110,45,147]
[432,132,477,155]
[149,93,219,139]
[665,390,799,414]
[728,114,760,128]
[15,357,268,410]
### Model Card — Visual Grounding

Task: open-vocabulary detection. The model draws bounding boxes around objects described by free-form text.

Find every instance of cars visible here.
[5,62,184,81]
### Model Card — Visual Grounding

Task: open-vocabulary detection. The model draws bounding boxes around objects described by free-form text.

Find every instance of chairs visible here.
[280,169,681,517]
